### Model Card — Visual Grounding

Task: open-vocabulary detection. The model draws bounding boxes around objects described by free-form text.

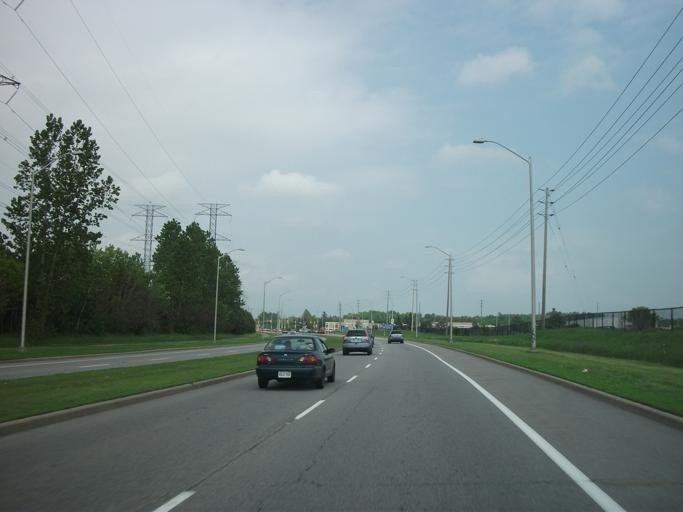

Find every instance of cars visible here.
[256,329,374,389]
[388,330,403,344]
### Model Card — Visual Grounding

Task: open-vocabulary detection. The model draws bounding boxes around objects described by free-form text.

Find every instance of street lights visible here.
[214,249,245,343]
[263,277,282,329]
[425,246,452,339]
[473,140,536,349]
[21,151,84,347]
